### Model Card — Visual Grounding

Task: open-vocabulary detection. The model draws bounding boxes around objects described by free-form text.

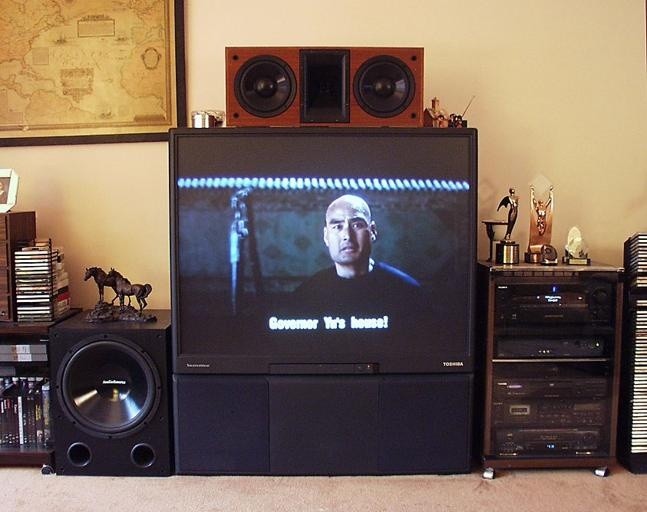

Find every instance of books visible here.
[0,343,54,448]
[14,239,72,322]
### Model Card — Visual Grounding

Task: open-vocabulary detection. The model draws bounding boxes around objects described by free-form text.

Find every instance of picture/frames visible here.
[0,0,188,147]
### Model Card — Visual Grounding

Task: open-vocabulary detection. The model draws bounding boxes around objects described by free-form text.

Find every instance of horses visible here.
[84,267,132,307]
[105,268,152,318]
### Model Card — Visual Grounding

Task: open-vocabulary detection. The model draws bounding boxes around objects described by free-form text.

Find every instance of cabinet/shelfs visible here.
[0,307,84,467]
[477,258,624,479]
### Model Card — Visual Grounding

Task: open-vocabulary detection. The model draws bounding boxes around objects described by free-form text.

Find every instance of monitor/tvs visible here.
[167,128,478,373]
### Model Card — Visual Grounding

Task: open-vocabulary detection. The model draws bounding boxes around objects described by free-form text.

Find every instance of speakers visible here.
[225,46,425,128]
[48,308,174,476]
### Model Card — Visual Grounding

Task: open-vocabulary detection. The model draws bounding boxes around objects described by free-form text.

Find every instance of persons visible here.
[274,194,434,305]
